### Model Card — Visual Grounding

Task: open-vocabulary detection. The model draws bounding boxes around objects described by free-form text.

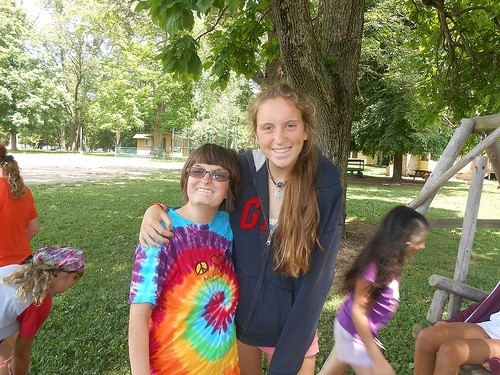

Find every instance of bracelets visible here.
[147,202,168,212]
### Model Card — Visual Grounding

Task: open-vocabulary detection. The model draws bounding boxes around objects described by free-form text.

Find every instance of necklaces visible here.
[265,158,292,199]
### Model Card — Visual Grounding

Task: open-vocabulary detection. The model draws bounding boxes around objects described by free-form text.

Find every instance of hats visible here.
[32,245,84,271]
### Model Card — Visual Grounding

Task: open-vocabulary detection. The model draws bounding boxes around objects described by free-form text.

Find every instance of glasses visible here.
[186,166,230,182]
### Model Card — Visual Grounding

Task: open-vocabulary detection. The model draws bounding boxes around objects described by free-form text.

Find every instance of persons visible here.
[0,136,39,268]
[137,80,344,375]
[129,143,240,375]
[413,273,500,375]
[315,205,429,375]
[0,242,86,375]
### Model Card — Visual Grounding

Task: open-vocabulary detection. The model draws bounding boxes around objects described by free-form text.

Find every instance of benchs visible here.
[409,174,423,178]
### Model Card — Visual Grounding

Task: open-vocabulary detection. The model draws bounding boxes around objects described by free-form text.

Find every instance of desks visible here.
[412,170,432,183]
